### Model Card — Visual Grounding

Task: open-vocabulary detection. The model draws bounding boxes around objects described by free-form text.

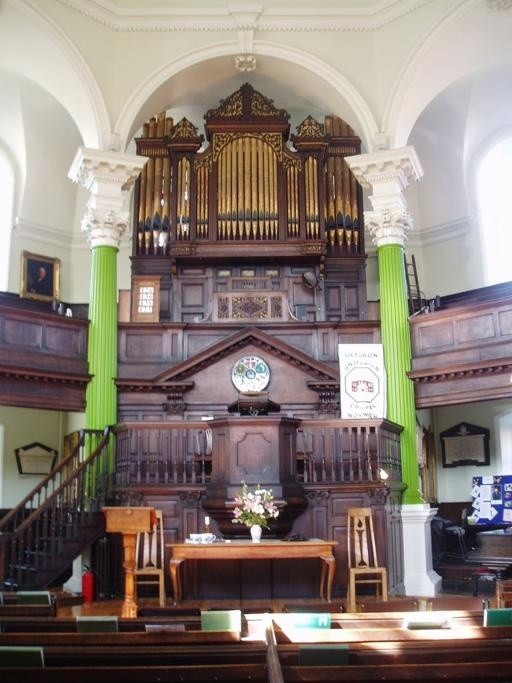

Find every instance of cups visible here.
[189,533,202,543]
[199,532,216,544]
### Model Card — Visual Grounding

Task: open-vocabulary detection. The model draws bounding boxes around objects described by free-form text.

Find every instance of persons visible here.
[30,267,50,295]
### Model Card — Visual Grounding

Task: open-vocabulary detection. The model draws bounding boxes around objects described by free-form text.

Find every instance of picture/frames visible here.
[19,248,59,303]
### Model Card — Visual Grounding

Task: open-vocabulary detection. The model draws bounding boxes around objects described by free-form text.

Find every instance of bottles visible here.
[202,515,212,533]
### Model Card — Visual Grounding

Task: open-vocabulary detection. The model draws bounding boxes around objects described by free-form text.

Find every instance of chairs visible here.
[346,507,389,607]
[129,508,166,610]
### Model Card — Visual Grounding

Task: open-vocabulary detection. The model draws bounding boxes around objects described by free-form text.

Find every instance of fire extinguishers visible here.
[82,564,100,604]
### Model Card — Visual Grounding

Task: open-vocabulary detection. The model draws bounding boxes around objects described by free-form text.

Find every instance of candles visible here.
[205,515,208,524]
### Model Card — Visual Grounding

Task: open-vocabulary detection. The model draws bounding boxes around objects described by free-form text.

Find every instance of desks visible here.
[164,540,340,607]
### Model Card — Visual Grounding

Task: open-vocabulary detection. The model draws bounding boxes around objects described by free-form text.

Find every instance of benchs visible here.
[2,578,512,683]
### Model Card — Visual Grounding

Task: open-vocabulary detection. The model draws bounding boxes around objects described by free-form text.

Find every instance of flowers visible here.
[230,478,285,530]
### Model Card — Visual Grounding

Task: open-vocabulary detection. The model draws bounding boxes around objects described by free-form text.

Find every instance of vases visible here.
[249,523,263,542]
